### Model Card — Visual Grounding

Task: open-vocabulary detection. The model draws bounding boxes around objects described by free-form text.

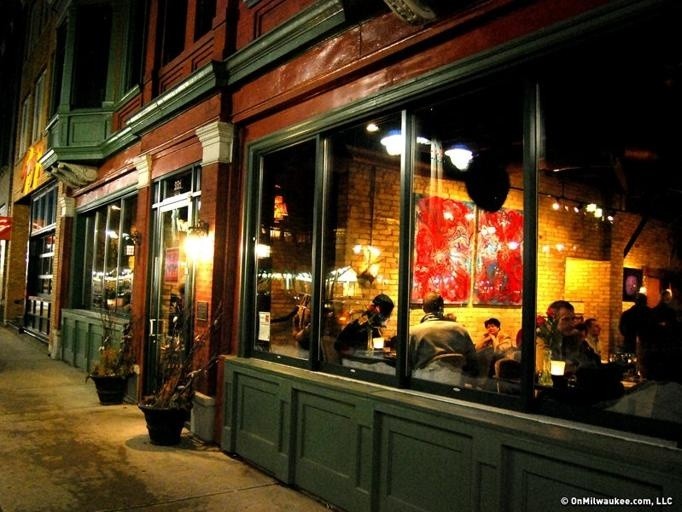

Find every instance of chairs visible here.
[424,353,524,409]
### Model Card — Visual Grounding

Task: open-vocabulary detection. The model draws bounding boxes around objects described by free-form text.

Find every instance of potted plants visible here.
[84,284,225,445]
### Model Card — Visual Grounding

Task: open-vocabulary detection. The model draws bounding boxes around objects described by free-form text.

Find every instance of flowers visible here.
[534,305,561,351]
[357,303,386,330]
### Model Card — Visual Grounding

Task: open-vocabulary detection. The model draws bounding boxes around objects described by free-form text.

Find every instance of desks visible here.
[338,347,397,367]
[491,374,543,398]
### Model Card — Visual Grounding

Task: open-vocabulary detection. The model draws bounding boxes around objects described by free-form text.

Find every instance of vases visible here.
[538,352,553,387]
[366,328,374,353]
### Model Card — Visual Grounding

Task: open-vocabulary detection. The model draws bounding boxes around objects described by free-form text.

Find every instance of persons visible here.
[409,290,481,378]
[652,288,679,324]
[617,293,655,353]
[333,294,395,364]
[175,283,186,313]
[475,300,603,378]
